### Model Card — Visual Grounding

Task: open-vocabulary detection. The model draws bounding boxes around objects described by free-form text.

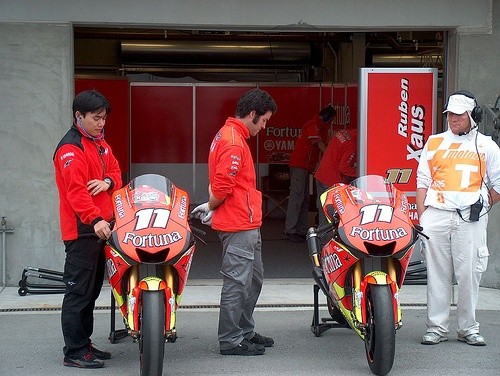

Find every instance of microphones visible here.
[458,122,479,136]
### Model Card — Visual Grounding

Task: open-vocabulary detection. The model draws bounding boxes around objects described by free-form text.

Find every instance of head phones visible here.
[443,90,483,123]
[323,113,329,122]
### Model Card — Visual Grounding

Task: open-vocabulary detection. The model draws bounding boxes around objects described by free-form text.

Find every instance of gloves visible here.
[192,202,210,220]
[202,210,214,225]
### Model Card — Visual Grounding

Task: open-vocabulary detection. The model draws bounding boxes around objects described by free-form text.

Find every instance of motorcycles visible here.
[103,172,209,376]
[304,173,430,376]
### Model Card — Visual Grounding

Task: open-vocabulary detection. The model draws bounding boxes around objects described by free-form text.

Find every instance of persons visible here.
[313,128,358,226]
[189,88,278,356]
[416,89,500,346]
[283,105,336,244]
[52,88,123,369]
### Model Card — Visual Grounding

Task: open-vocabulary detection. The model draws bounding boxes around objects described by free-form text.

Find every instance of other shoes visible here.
[284,231,305,243]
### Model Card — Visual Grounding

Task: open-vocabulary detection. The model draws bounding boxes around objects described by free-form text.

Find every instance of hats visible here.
[443,94,474,115]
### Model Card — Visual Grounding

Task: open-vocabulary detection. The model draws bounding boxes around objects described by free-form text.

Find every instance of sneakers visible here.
[88,345,111,360]
[422,332,448,344]
[457,333,486,345]
[250,332,274,346]
[220,338,265,355]
[63,353,104,368]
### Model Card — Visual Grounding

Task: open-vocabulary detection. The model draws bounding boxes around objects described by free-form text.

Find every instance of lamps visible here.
[121,39,310,54]
[372,55,442,64]
